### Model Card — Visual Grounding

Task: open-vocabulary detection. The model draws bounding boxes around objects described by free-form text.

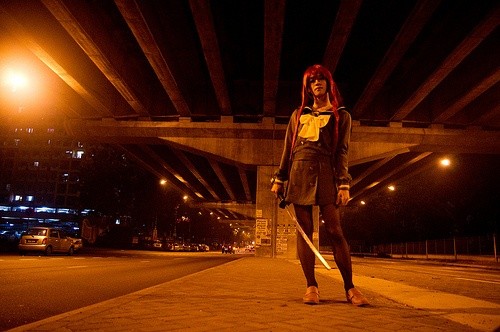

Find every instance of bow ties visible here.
[298,114,331,143]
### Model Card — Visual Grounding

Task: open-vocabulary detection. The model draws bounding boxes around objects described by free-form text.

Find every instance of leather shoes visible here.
[345,287,371,307]
[302,285,319,304]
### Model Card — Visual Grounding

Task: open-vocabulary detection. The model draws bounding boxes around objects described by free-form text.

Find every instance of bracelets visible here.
[270,178,284,186]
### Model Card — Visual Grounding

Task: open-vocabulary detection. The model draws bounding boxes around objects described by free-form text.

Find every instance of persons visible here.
[270,64,370,306]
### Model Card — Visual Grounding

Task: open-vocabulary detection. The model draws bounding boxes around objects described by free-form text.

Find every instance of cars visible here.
[17,226,75,256]
[221,243,235,255]
[233,247,239,251]
[147,240,210,252]
[245,245,255,251]
[68,234,83,254]
